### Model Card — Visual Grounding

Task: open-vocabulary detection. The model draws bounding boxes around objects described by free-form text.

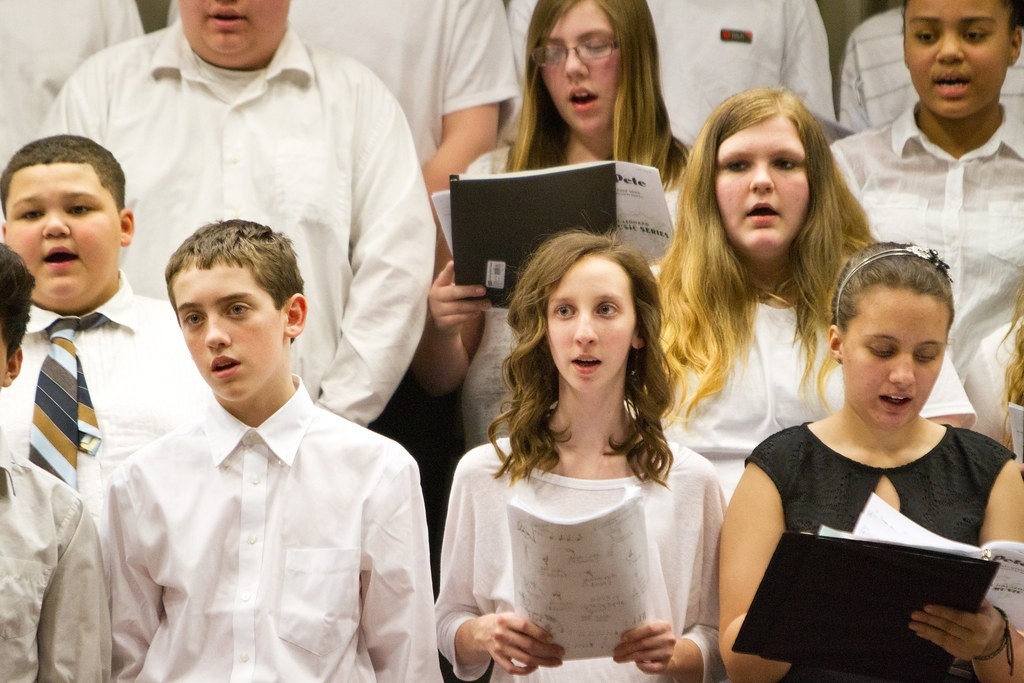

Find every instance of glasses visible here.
[531,38,620,67]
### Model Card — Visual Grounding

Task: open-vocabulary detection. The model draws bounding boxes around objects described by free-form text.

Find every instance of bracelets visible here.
[973,605,1014,676]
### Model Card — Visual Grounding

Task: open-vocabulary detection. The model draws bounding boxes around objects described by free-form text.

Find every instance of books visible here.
[729,495,1024,683]
[506,485,648,660]
[431,160,674,309]
[1009,402,1024,465]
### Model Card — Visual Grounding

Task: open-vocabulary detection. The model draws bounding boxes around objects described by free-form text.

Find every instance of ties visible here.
[29,312,109,493]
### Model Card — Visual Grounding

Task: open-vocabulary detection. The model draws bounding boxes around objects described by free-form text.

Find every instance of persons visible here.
[658,87,978,499]
[1,1,143,174]
[414,0,1024,448]
[964,277,1024,453]
[434,229,727,683]
[166,0,517,427]
[0,243,112,683]
[98,219,444,683]
[0,134,212,530]
[45,0,436,430]
[717,242,1024,683]
